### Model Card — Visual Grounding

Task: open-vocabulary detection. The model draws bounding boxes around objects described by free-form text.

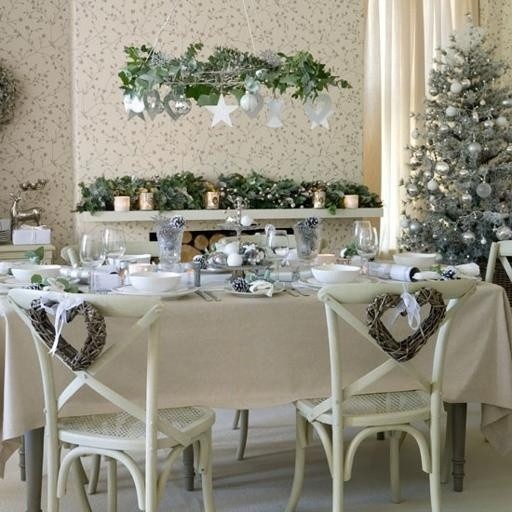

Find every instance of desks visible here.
[0,244,56,266]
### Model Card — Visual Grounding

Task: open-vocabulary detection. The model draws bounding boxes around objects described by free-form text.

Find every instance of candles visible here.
[114,196,130,212]
[204,191,219,210]
[139,192,154,210]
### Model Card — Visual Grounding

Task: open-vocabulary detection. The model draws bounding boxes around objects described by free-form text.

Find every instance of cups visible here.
[114,192,155,211]
[207,192,220,210]
[389,265,420,282]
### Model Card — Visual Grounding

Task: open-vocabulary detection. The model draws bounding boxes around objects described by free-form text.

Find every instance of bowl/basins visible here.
[129,271,179,291]
[311,264,360,283]
[393,251,438,269]
[10,263,60,284]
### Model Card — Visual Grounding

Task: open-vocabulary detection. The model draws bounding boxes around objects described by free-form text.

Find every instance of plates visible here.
[0,273,80,288]
[113,284,199,298]
[224,283,285,296]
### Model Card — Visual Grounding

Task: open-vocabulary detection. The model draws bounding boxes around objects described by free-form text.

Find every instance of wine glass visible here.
[267,230,289,286]
[80,226,126,293]
[353,220,381,281]
